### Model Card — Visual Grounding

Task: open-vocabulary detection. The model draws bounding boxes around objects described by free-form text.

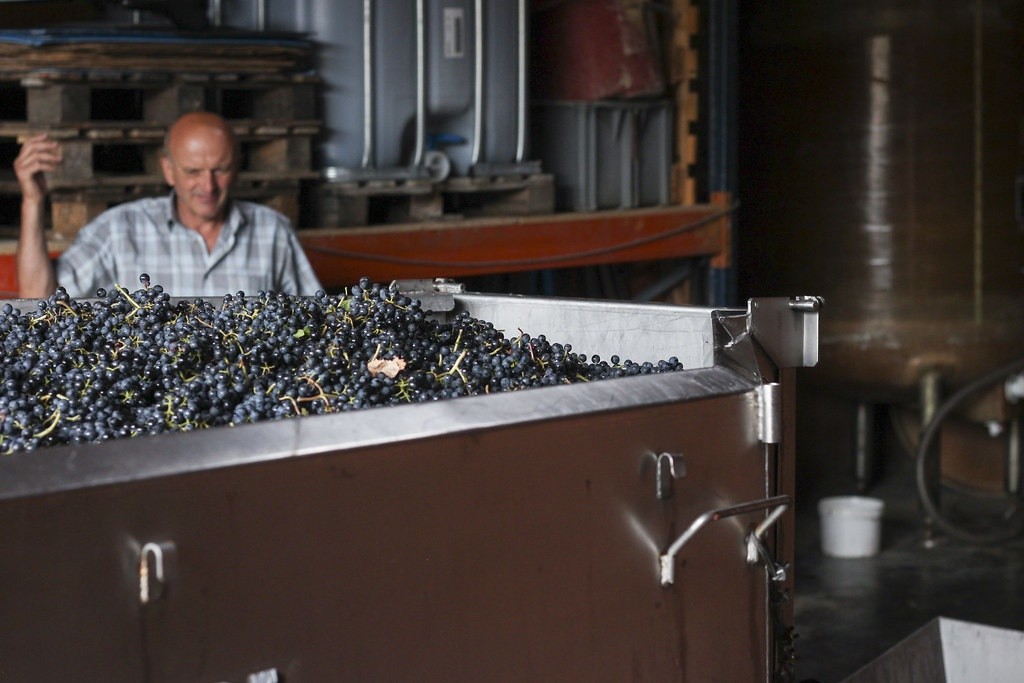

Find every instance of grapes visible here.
[0,274,684,455]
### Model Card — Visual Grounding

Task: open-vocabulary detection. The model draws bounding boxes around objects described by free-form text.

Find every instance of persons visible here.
[13,111,325,299]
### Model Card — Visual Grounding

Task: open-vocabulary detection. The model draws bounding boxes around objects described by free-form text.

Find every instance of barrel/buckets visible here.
[817,497,884,559]
